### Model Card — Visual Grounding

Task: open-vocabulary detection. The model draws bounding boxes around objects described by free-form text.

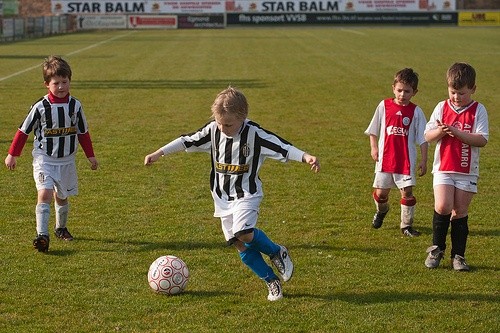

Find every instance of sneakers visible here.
[450,254,470,271]
[266,279,283,301]
[372,210,389,228]
[402,226,419,237]
[271,245,293,282]
[424,245,445,268]
[33,234,49,253]
[54,227,73,241]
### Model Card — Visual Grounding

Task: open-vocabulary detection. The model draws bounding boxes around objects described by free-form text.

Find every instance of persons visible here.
[364,67,429,237]
[144,88,320,301]
[424,63,490,270]
[5,55,97,251]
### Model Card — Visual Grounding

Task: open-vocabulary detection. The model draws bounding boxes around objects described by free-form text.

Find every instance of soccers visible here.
[147,255,192,295]
